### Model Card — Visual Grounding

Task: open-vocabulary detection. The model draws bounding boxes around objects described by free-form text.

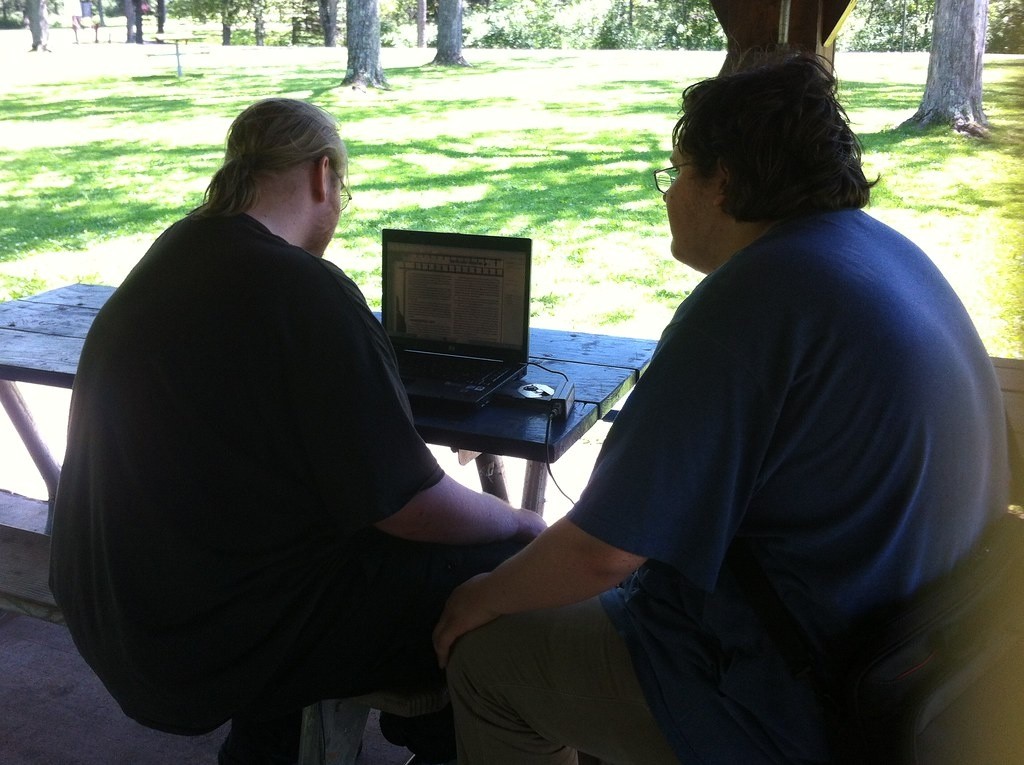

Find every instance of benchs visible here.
[0,504,449,765]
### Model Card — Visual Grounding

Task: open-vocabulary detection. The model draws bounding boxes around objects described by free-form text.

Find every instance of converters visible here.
[550,381,575,421]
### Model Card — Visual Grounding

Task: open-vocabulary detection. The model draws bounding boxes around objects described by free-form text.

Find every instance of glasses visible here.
[313,154,352,211]
[652,162,695,194]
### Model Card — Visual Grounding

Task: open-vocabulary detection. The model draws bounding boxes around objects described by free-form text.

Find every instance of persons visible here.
[431,49,1006,763]
[42,97,550,763]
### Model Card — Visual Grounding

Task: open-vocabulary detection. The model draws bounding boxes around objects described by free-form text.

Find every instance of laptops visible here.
[380,228,558,413]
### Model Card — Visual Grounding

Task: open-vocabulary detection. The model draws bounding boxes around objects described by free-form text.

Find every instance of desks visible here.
[154,32,206,77]
[0,282,660,523]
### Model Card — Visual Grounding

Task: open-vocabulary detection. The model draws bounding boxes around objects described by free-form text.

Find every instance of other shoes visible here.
[378,709,455,763]
